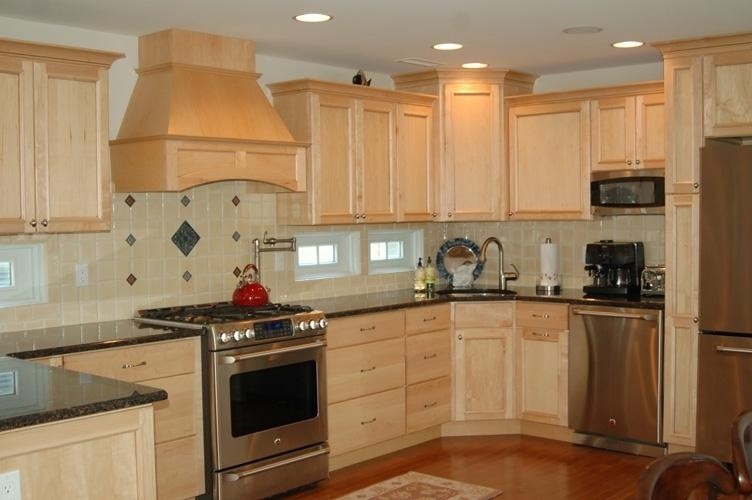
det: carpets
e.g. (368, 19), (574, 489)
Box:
(333, 469), (504, 500)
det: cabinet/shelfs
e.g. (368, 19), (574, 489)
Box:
(592, 85), (665, 173)
(266, 78), (395, 223)
(395, 90), (437, 223)
(57, 337), (205, 500)
(406, 303), (450, 449)
(442, 68), (539, 223)
(0, 39), (127, 235)
(514, 302), (569, 442)
(663, 50), (701, 447)
(507, 87), (613, 221)
(324, 301), (405, 464)
(451, 300), (513, 439)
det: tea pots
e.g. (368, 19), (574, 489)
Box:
(231, 265), (268, 307)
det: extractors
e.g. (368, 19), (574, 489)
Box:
(111, 31), (310, 196)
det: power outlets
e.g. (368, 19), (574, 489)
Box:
(1, 468), (24, 500)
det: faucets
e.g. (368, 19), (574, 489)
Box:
(480, 237), (520, 290)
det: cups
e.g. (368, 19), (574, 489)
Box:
(639, 264), (664, 297)
(424, 256), (436, 286)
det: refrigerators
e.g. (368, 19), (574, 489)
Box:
(695, 142), (752, 468)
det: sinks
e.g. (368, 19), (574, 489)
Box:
(437, 287), (516, 299)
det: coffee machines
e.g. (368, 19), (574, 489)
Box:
(582, 238), (643, 302)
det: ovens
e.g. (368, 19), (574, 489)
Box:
(195, 334), (330, 500)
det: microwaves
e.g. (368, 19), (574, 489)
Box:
(590, 170), (664, 215)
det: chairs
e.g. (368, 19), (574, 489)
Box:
(639, 451), (742, 499)
(732, 408), (752, 500)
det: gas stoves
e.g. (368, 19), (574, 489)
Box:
(132, 293), (331, 352)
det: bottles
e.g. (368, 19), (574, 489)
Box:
(412, 257), (426, 291)
(541, 237), (559, 286)
